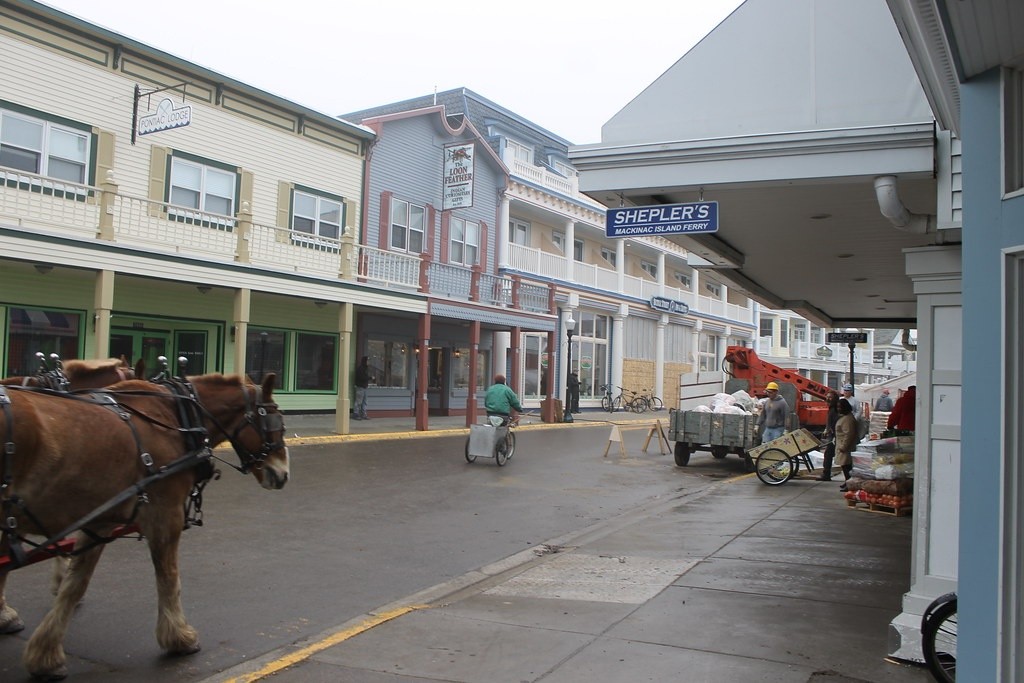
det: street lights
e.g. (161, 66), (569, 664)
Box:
(564, 314), (576, 423)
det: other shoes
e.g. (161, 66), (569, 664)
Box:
(815, 476), (831, 481)
(575, 408), (582, 413)
(840, 486), (848, 492)
(840, 483), (847, 488)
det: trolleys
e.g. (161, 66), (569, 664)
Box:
(746, 428), (833, 486)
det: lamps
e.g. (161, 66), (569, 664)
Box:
(230, 326), (235, 343)
(315, 302), (327, 309)
(35, 265), (53, 275)
(197, 287), (211, 294)
(460, 323), (471, 328)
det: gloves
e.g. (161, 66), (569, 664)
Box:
(783, 429), (789, 436)
(753, 425), (760, 435)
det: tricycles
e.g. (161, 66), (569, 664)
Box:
(465, 416), (517, 466)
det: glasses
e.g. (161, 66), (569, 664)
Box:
(766, 390), (773, 394)
(844, 389), (850, 392)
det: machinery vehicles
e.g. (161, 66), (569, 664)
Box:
(722, 345), (871, 440)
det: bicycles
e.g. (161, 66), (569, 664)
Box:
(613, 385), (663, 413)
(599, 383), (614, 414)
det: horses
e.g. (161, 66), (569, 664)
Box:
(0, 355), (291, 680)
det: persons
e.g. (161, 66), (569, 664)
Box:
(484, 375), (522, 454)
(815, 390), (844, 481)
(570, 368), (582, 414)
(835, 399), (859, 492)
(754, 382), (792, 474)
(875, 389), (893, 412)
(353, 356), (375, 420)
(841, 383), (862, 444)
(887, 385), (915, 435)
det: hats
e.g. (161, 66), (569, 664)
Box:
(573, 368), (579, 371)
(883, 389), (890, 394)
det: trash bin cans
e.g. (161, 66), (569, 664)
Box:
(540, 398), (563, 423)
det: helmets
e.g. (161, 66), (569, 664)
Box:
(843, 384), (852, 390)
(765, 382), (779, 391)
